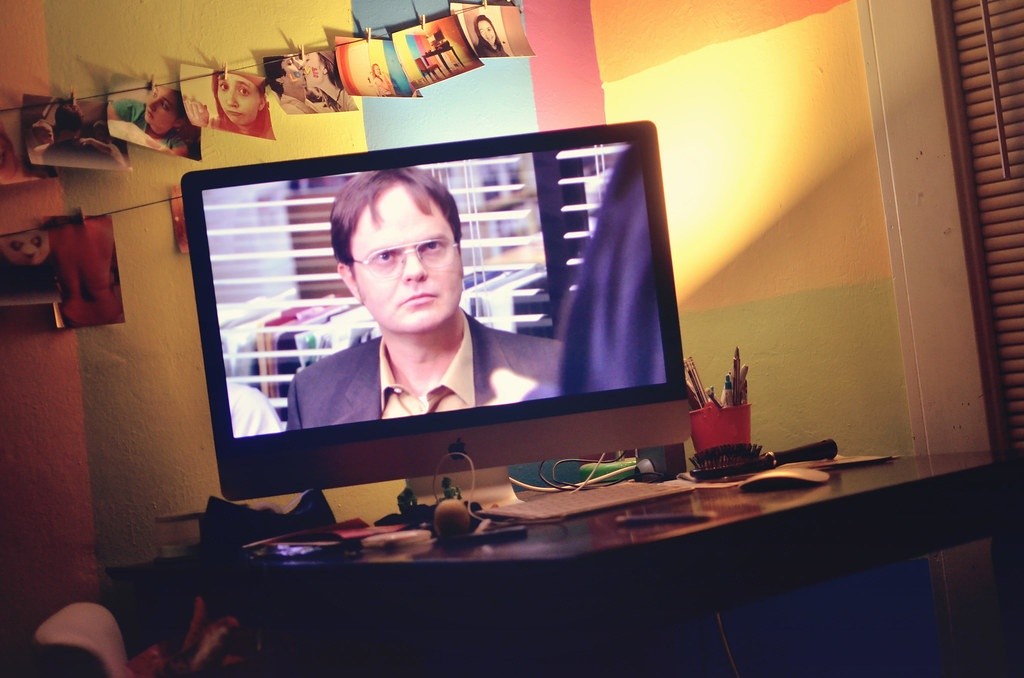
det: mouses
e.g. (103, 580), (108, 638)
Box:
(739, 467), (828, 493)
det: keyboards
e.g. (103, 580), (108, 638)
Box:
(482, 482), (697, 521)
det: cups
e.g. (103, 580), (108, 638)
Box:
(686, 404), (751, 459)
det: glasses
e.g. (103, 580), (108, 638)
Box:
(353, 239), (458, 281)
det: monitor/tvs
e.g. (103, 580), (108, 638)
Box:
(184, 127), (691, 511)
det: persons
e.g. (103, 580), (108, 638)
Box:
(264, 52), (359, 114)
(107, 85), (188, 157)
(366, 63), (395, 97)
(183, 70), (276, 140)
(474, 15), (509, 57)
(286, 167), (566, 434)
(31, 104), (129, 170)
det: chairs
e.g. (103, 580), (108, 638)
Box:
(34, 602), (128, 678)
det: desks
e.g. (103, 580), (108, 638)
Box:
(103, 452), (1024, 678)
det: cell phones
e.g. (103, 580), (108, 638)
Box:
(433, 525), (527, 550)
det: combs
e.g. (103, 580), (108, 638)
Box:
(686, 439), (838, 479)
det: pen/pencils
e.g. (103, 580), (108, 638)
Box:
(684, 345), (749, 410)
(432, 526), (529, 547)
(615, 513), (712, 526)
